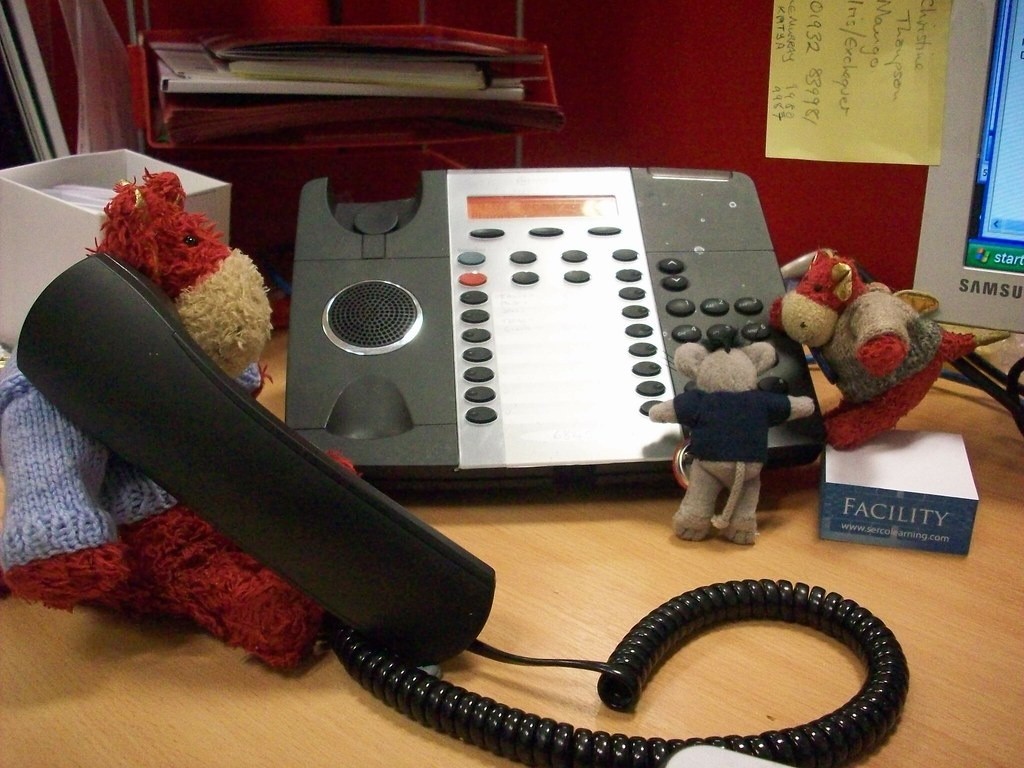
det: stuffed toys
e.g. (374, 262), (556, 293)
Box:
(0, 168), (359, 671)
(649, 342), (815, 544)
(769, 250), (1008, 450)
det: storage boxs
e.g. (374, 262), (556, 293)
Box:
(0, 148), (234, 350)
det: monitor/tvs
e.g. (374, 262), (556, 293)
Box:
(912, 1), (1024, 331)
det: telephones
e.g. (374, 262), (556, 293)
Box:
(14, 251), (496, 664)
